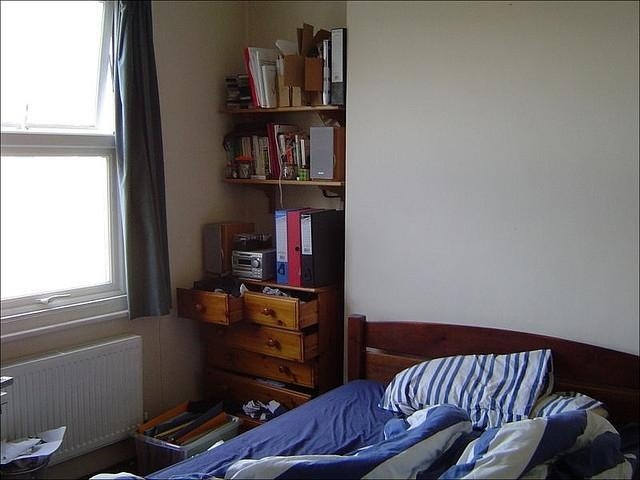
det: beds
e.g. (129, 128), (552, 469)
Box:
(90, 314), (640, 480)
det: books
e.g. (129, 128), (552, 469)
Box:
(221, 44), (310, 180)
(139, 399), (234, 447)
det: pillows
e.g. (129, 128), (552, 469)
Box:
(531, 391), (608, 425)
(378, 349), (554, 431)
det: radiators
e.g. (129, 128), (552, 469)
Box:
(0, 333), (143, 466)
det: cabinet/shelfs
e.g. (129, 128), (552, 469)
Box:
(178, 279), (318, 435)
(216, 104), (344, 187)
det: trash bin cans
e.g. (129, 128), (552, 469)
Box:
(0, 437), (51, 480)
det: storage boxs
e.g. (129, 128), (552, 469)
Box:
(131, 414), (243, 473)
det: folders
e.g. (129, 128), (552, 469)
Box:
(275, 209), (345, 288)
